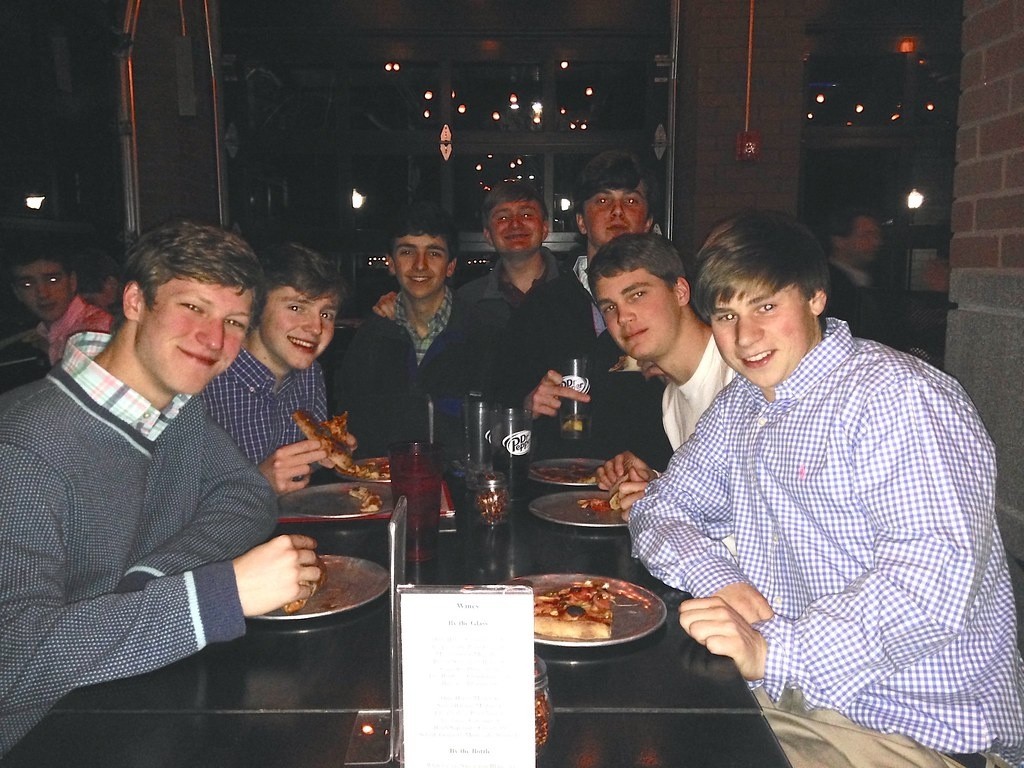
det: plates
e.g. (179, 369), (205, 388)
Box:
(282, 482), (395, 519)
(500, 572), (667, 648)
(248, 555), (389, 621)
(527, 458), (607, 486)
(335, 455), (391, 482)
(528, 490), (628, 528)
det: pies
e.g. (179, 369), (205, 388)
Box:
(608, 355), (640, 371)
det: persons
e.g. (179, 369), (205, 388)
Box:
(77, 244), (120, 305)
(210, 237), (348, 498)
(826, 211), (894, 344)
(590, 233), (734, 521)
(629, 212), (1024, 767)
(371, 150), (674, 472)
(333, 204), (494, 456)
(0, 225), (321, 754)
(3, 234), (117, 372)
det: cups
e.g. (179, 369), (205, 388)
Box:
(462, 400), (502, 476)
(557, 355), (594, 439)
(386, 441), (444, 560)
(489, 408), (536, 493)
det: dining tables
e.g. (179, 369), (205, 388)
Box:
(0, 457), (792, 768)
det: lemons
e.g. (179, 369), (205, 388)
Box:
(562, 421), (582, 431)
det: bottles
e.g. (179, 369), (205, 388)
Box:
(474, 475), (514, 526)
(534, 654), (552, 756)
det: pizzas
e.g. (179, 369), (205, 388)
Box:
(528, 465), (598, 482)
(534, 586), (613, 640)
(348, 485), (382, 513)
(284, 556), (328, 613)
(290, 410), (355, 473)
(337, 456), (390, 480)
